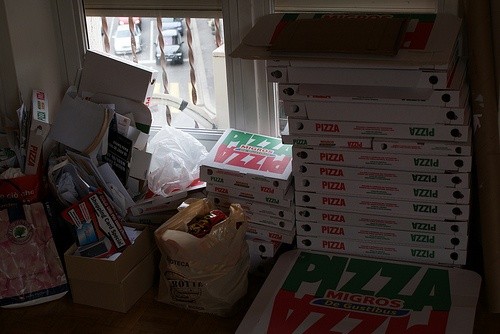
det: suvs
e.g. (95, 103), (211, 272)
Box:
(152, 30), (185, 65)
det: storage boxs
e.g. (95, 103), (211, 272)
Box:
(0, 12), (478, 334)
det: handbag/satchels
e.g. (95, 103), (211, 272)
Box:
(0, 180), (69, 309)
(154, 197), (249, 315)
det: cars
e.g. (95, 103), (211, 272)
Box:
(118, 17), (142, 26)
(111, 23), (142, 54)
(161, 18), (184, 36)
